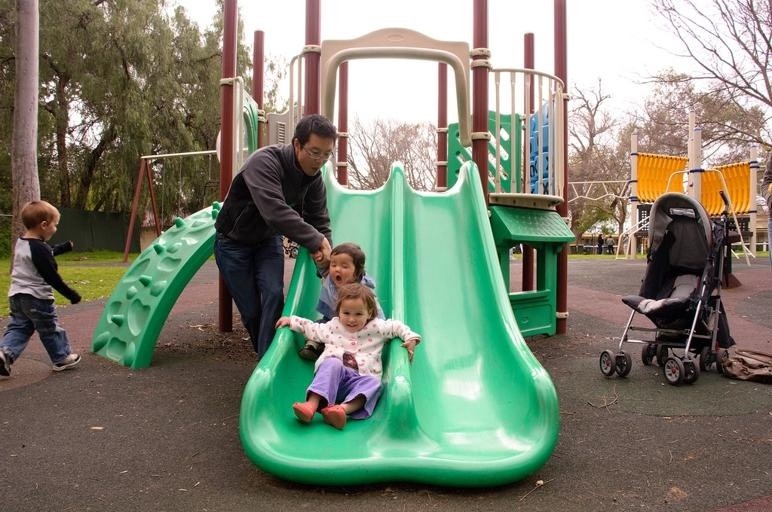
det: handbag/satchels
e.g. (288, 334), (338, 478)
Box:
(722, 347), (772, 381)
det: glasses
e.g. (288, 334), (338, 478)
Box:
(301, 144), (335, 160)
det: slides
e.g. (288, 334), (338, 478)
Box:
(239, 159), (560, 490)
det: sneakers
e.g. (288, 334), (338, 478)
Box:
(298, 343), (325, 360)
(0, 348), (12, 376)
(292, 401), (347, 429)
(52, 354), (81, 371)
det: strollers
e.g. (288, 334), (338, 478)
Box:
(601, 191), (734, 387)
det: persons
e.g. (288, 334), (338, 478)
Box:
(214, 113), (340, 363)
(607, 235), (615, 255)
(597, 234), (604, 254)
(297, 242), (387, 362)
(760, 150), (771, 272)
(0, 200), (84, 378)
(274, 282), (422, 431)
(623, 233), (628, 256)
